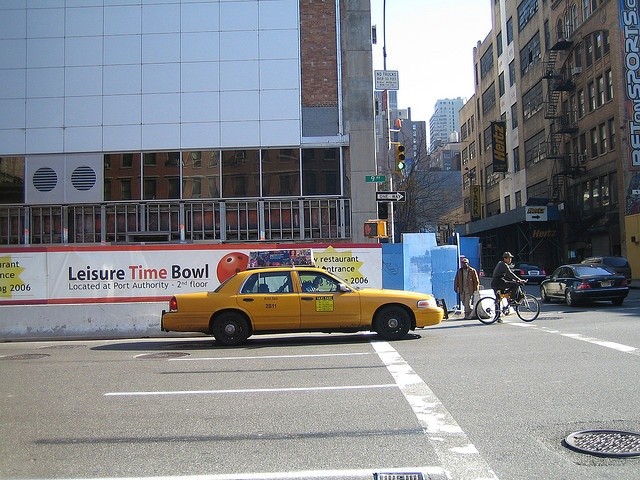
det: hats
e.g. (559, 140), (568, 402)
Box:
(502, 251), (514, 258)
(461, 258), (469, 264)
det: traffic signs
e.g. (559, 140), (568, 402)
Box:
(375, 191), (406, 203)
(364, 175), (386, 182)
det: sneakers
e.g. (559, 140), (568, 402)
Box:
(510, 299), (520, 306)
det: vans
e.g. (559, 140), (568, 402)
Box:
(580, 256), (632, 285)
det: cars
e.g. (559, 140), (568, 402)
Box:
(161, 267), (444, 346)
(512, 262), (546, 285)
(539, 263), (629, 307)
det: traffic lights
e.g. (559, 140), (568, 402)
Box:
(395, 145), (406, 173)
(363, 219), (387, 239)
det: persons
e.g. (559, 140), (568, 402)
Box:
(302, 276), (323, 292)
(453, 258), (480, 320)
(258, 282), (289, 293)
(491, 252), (526, 323)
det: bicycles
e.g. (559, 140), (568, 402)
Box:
(475, 279), (540, 325)
